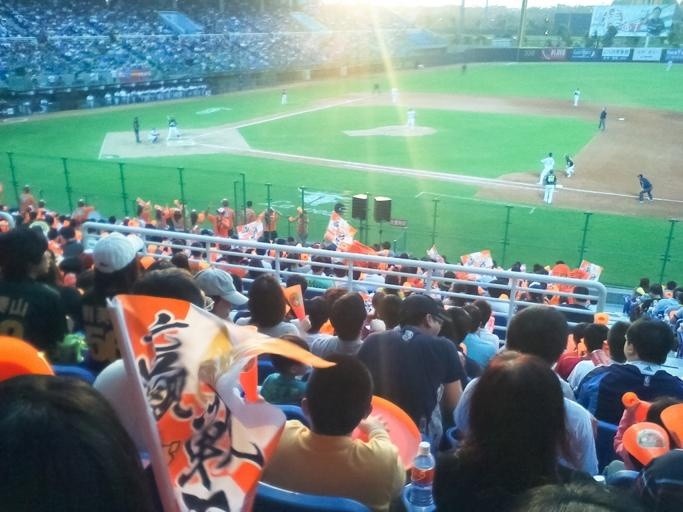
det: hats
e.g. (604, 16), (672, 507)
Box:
(193, 266), (249, 306)
(93, 231), (143, 273)
(398, 294), (453, 325)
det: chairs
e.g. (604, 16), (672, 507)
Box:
(45, 357), (646, 512)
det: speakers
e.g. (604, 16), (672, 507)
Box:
(374, 196), (391, 222)
(352, 194), (368, 221)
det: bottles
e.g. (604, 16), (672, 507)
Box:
(408, 441), (436, 506)
(484, 311), (495, 333)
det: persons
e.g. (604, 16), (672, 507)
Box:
(1, 186), (683, 512)
(597, 108), (607, 130)
(573, 88), (581, 106)
(565, 155), (575, 177)
(638, 175), (653, 204)
(148, 128), (160, 144)
(538, 153), (554, 185)
(406, 109), (415, 129)
(166, 116), (180, 141)
(642, 6), (666, 38)
(1, 0), (466, 117)
(667, 57), (673, 71)
(544, 170), (556, 203)
(133, 117), (141, 143)
